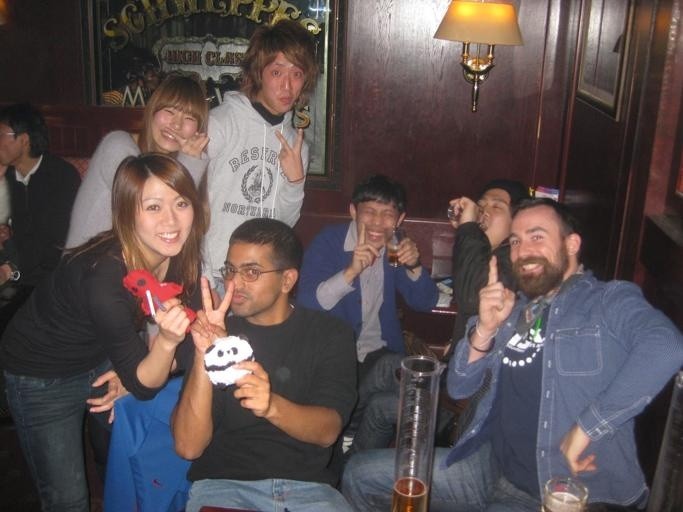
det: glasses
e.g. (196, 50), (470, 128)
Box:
(219, 266), (287, 282)
(1, 132), (17, 139)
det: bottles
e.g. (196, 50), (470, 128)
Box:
(390, 355), (442, 512)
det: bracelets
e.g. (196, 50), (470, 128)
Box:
(288, 174), (304, 185)
(6, 260), (21, 283)
(467, 326), (497, 356)
(7, 224), (14, 242)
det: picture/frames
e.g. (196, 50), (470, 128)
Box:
(575, 1), (636, 123)
(80, 1), (349, 192)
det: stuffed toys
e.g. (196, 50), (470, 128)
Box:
(119, 268), (198, 335)
(202, 333), (257, 394)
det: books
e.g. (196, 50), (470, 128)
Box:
(535, 185), (560, 203)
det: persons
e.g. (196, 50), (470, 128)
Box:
(339, 177), (530, 472)
(338, 197), (683, 510)
(0, 162), (13, 244)
(168, 217), (360, 512)
(293, 175), (441, 389)
(0, 103), (82, 328)
(195, 18), (324, 316)
(59, 70), (212, 265)
(0, 152), (208, 512)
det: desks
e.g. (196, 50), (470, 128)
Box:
(398, 277), (459, 351)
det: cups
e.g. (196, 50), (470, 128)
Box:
(447, 205), (489, 232)
(385, 227), (405, 267)
(543, 477), (589, 512)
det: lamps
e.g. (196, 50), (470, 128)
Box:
(433, 0), (525, 112)
